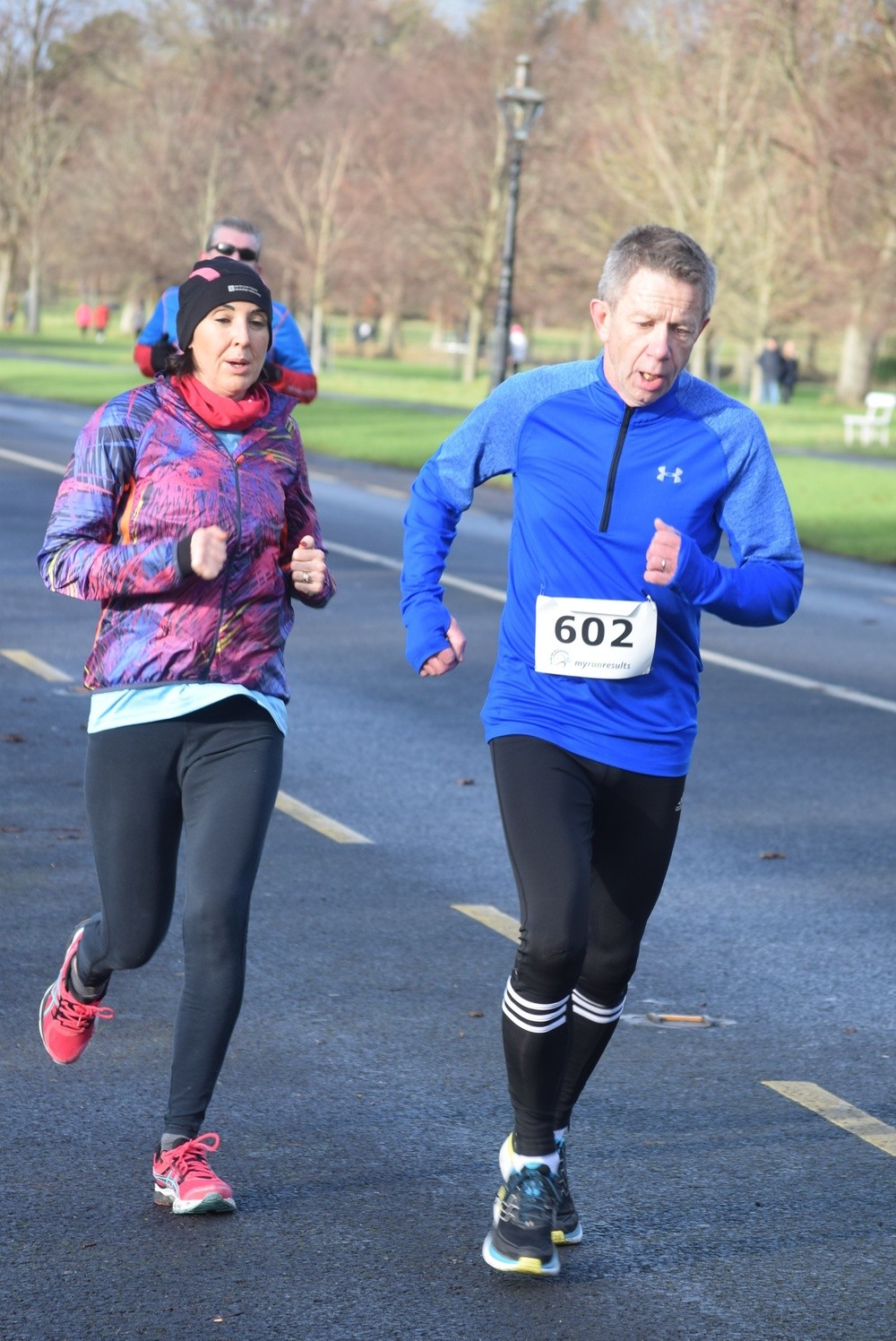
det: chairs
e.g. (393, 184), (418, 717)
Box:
(841, 392), (895, 448)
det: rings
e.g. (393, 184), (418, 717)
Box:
(302, 571), (309, 583)
(661, 559), (666, 572)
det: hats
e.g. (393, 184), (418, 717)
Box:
(177, 256), (272, 355)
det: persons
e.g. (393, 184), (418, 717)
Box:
(398, 221), (805, 1279)
(753, 330), (799, 404)
(506, 316), (529, 377)
(134, 217), (318, 424)
(76, 298), (109, 343)
(35, 257), (340, 1217)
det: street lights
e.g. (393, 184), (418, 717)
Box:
(489, 51), (544, 391)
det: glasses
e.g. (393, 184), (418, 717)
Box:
(208, 242), (257, 261)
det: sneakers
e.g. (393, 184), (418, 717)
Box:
(499, 1131), (582, 1245)
(153, 1133), (236, 1214)
(483, 1162), (560, 1274)
(39, 918), (114, 1065)
